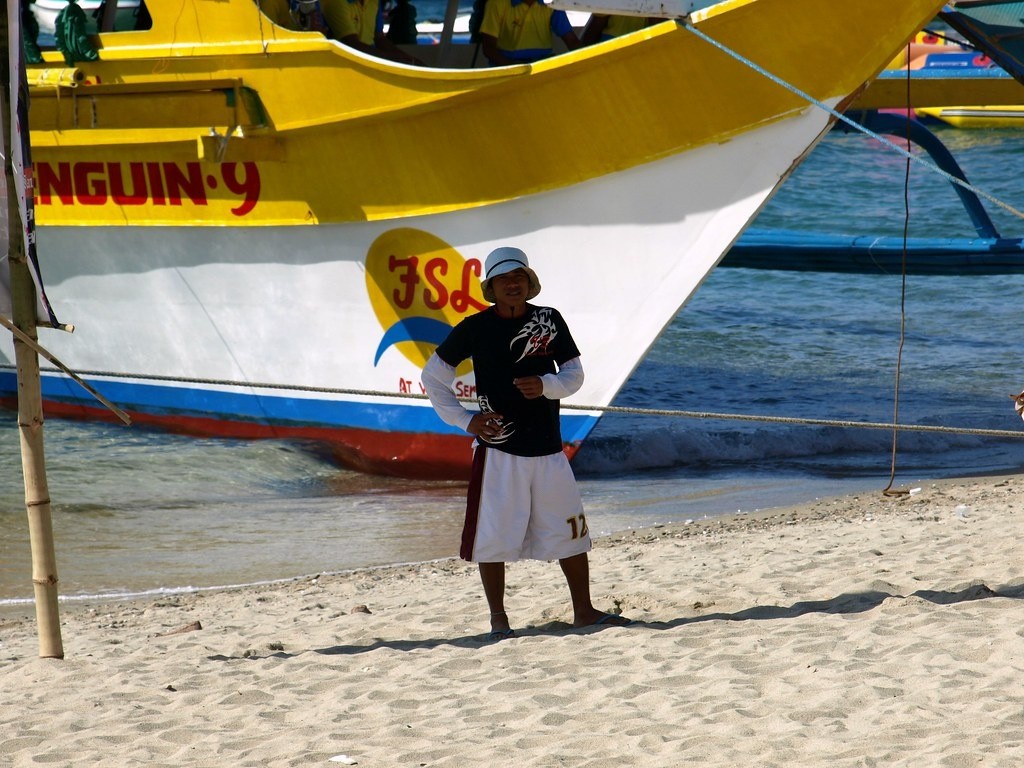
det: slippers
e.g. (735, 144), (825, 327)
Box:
(487, 629), (514, 643)
(596, 613), (635, 626)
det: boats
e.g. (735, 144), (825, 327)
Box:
(1, 1), (957, 482)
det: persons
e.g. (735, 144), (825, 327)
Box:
(470, 0), (488, 43)
(479, 0), (582, 65)
(21, 0), (39, 40)
(257, 0), (398, 58)
(422, 247), (632, 641)
(388, 0), (417, 44)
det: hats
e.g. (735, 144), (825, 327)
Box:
(480, 247), (541, 303)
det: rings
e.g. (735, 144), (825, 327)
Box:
(486, 421), (489, 425)
